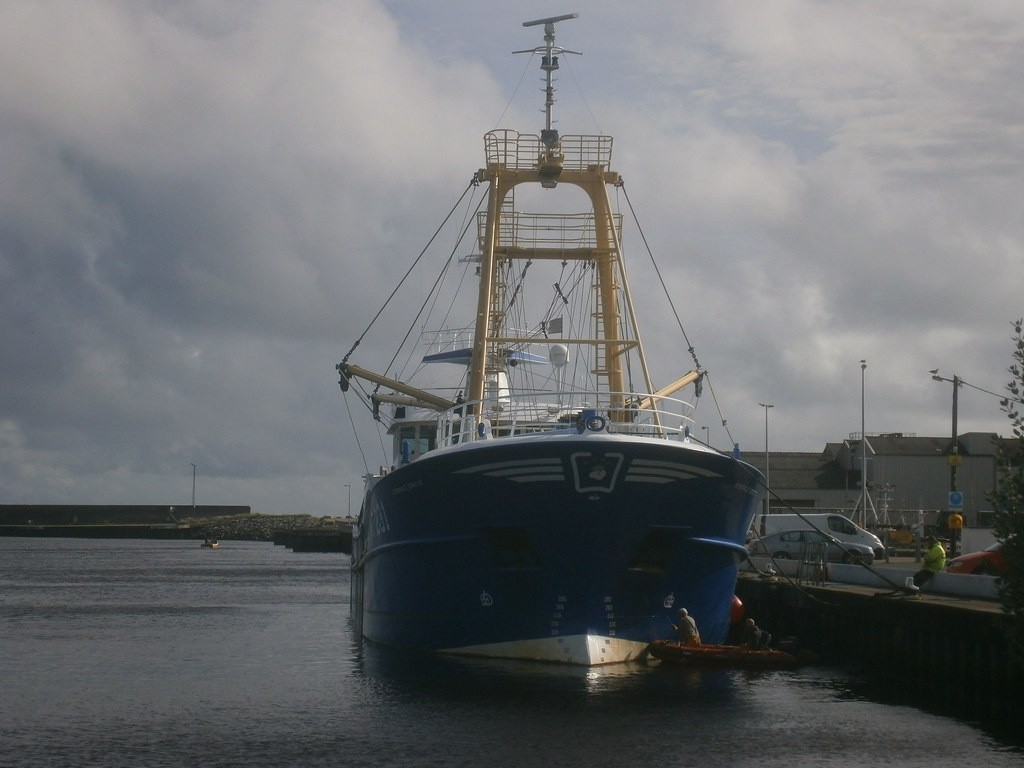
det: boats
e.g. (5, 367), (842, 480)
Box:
(646, 639), (797, 669)
(201, 539), (219, 548)
(333, 13), (768, 668)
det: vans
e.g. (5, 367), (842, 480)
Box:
(755, 512), (885, 559)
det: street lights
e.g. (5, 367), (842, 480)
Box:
(702, 426), (710, 449)
(192, 463), (196, 507)
(759, 403), (774, 513)
(861, 364), (867, 528)
(930, 374), (959, 491)
(345, 484), (350, 526)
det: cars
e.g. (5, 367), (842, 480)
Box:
(945, 533), (1019, 575)
(748, 529), (875, 565)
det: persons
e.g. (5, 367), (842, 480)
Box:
(670, 608), (765, 651)
(911, 535), (946, 587)
